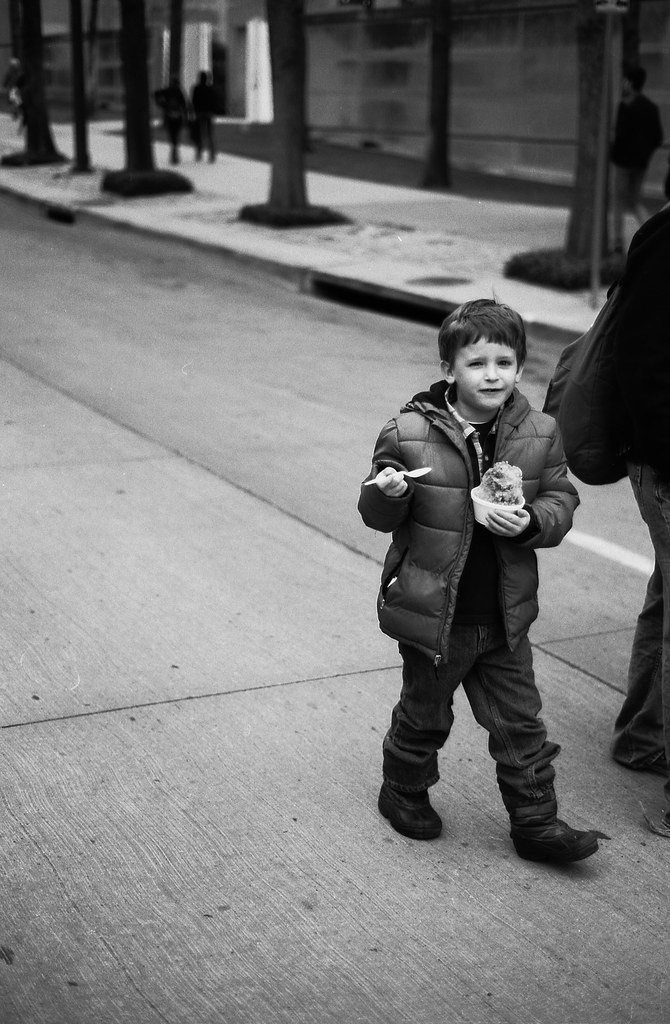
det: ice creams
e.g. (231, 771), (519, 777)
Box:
(471, 460), (525, 525)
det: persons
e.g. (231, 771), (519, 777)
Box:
(357, 300), (598, 863)
(610, 172), (670, 824)
(190, 71), (216, 162)
(615, 64), (663, 254)
(154, 82), (186, 163)
(4, 58), (27, 134)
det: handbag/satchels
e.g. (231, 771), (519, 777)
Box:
(542, 280), (630, 485)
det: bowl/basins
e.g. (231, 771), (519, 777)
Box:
(470, 486), (526, 526)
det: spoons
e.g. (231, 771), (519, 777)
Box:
(364, 466), (432, 487)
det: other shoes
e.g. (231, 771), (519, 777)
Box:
(661, 812), (670, 830)
(647, 754), (670, 777)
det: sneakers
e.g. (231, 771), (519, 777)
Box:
(379, 781), (442, 840)
(513, 825), (599, 864)
